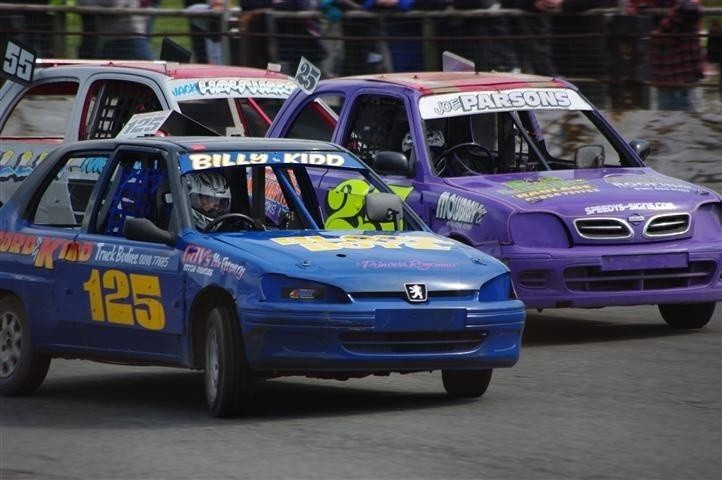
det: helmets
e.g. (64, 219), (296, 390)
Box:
(402, 128), (445, 152)
(183, 173), (233, 231)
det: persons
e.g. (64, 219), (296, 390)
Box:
(0, 1), (162, 58)
(183, 0), (721, 110)
(168, 171), (267, 233)
(401, 126), (456, 177)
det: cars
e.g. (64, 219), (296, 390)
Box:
(1, 58), (377, 210)
(245, 72), (722, 330)
(0, 135), (525, 421)
